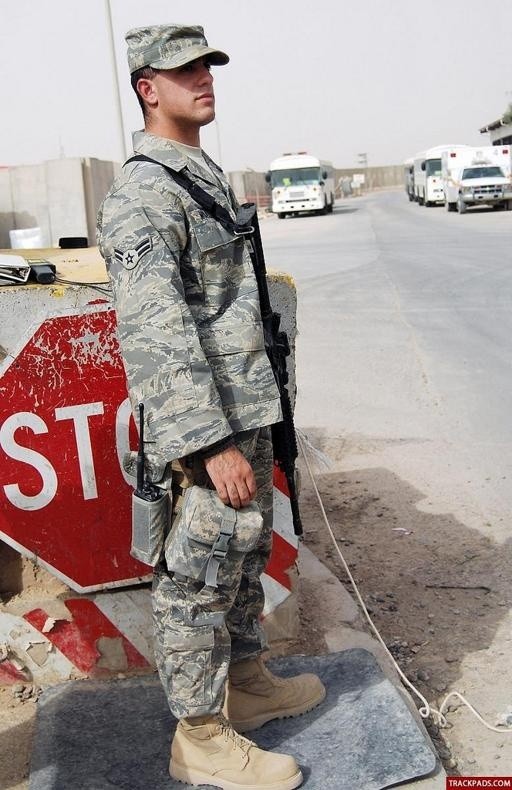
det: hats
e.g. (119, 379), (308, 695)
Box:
(124, 25), (230, 76)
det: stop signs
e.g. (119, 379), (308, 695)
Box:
(0, 307), (152, 600)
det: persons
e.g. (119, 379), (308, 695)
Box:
(96, 25), (328, 790)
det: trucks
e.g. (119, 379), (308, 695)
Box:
(402, 142), (512, 217)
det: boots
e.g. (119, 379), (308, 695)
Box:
(168, 711), (303, 790)
(223, 654), (327, 733)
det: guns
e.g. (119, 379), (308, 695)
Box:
(236, 203), (304, 535)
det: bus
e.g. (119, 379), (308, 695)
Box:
(264, 151), (340, 219)
